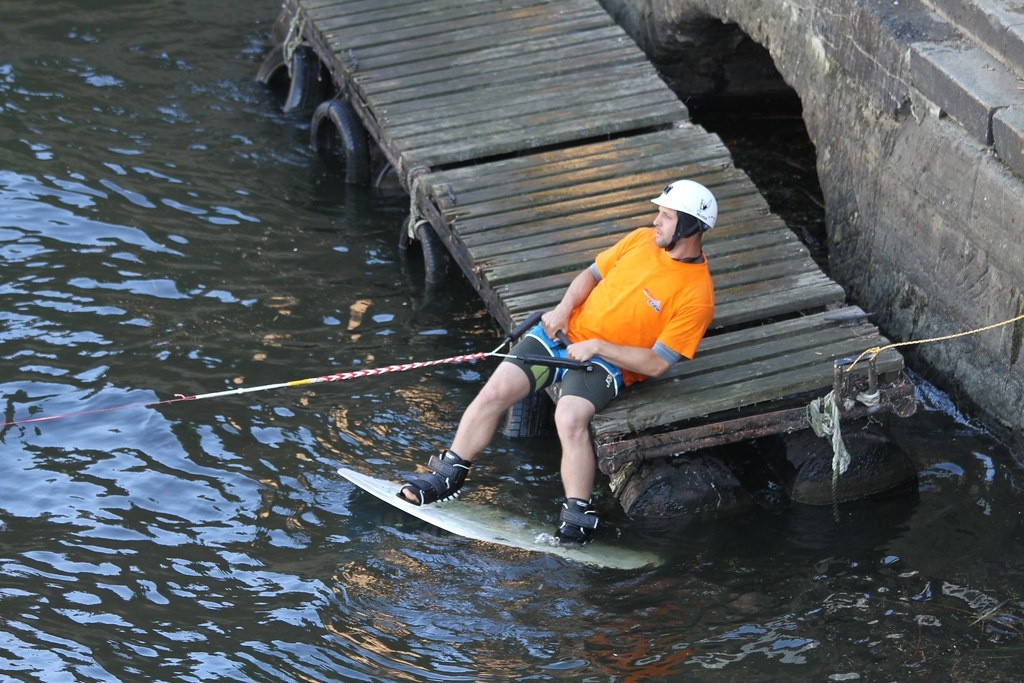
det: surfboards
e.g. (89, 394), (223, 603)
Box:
(335, 464), (665, 572)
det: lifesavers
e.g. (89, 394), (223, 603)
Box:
(253, 39), (320, 117)
(399, 214), (451, 287)
(306, 95), (372, 189)
(503, 385), (541, 441)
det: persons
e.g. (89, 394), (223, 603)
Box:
(399, 180), (719, 544)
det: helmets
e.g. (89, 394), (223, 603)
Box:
(651, 179), (718, 228)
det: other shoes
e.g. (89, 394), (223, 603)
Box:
(552, 497), (600, 548)
(396, 449), (472, 509)
